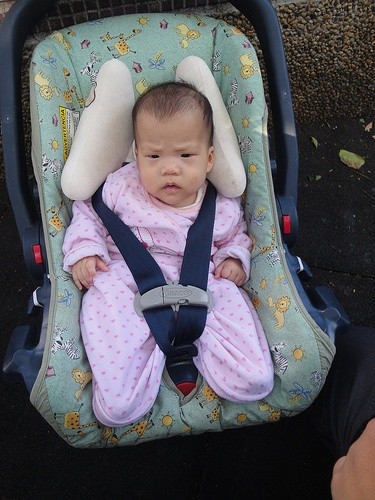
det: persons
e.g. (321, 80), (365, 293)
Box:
(61, 80), (276, 426)
(328, 419), (375, 499)
